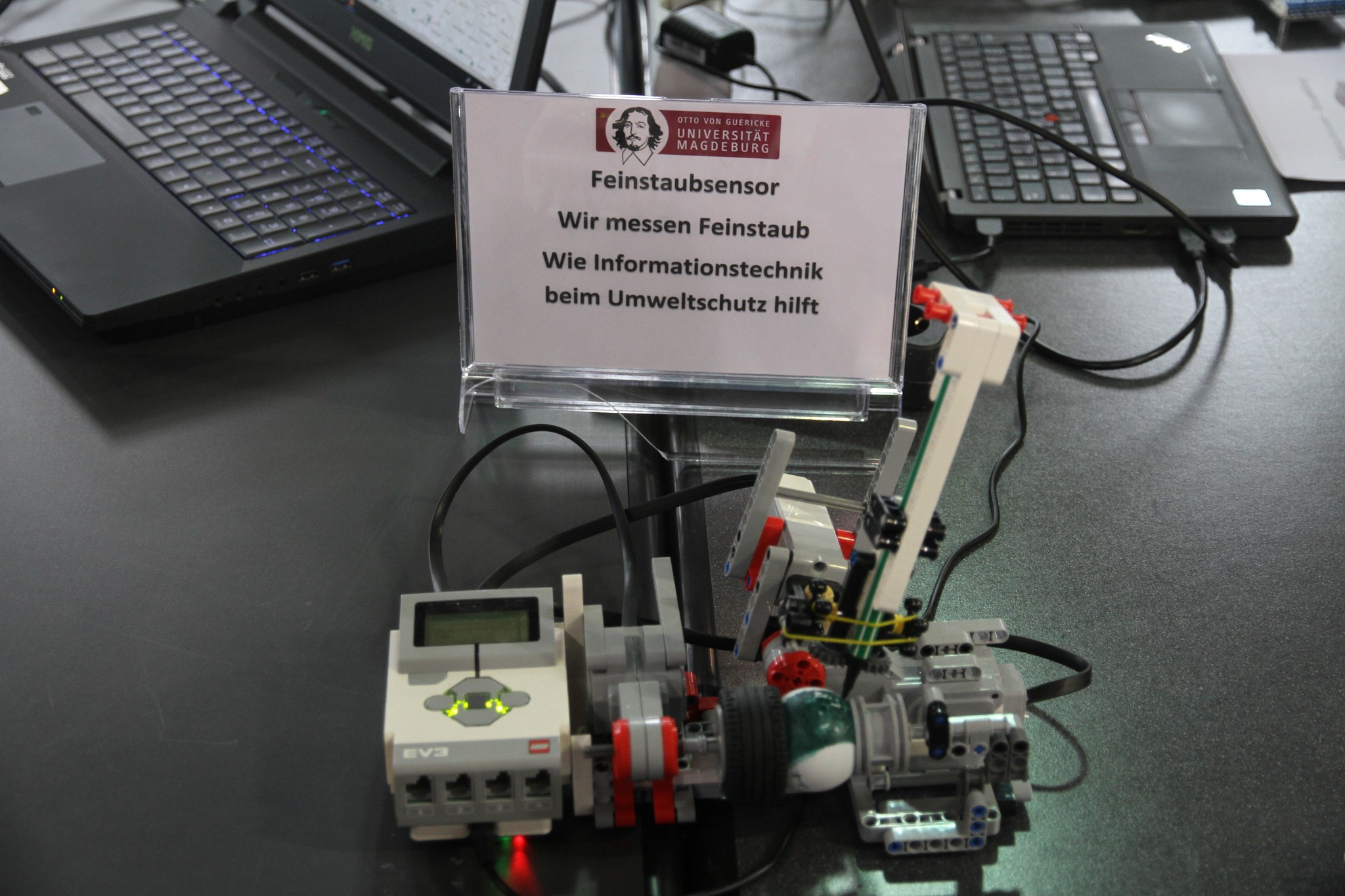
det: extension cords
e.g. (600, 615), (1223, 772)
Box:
(646, 1), (731, 102)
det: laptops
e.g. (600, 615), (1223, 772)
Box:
(0, 0), (558, 334)
(849, 0), (1302, 240)
